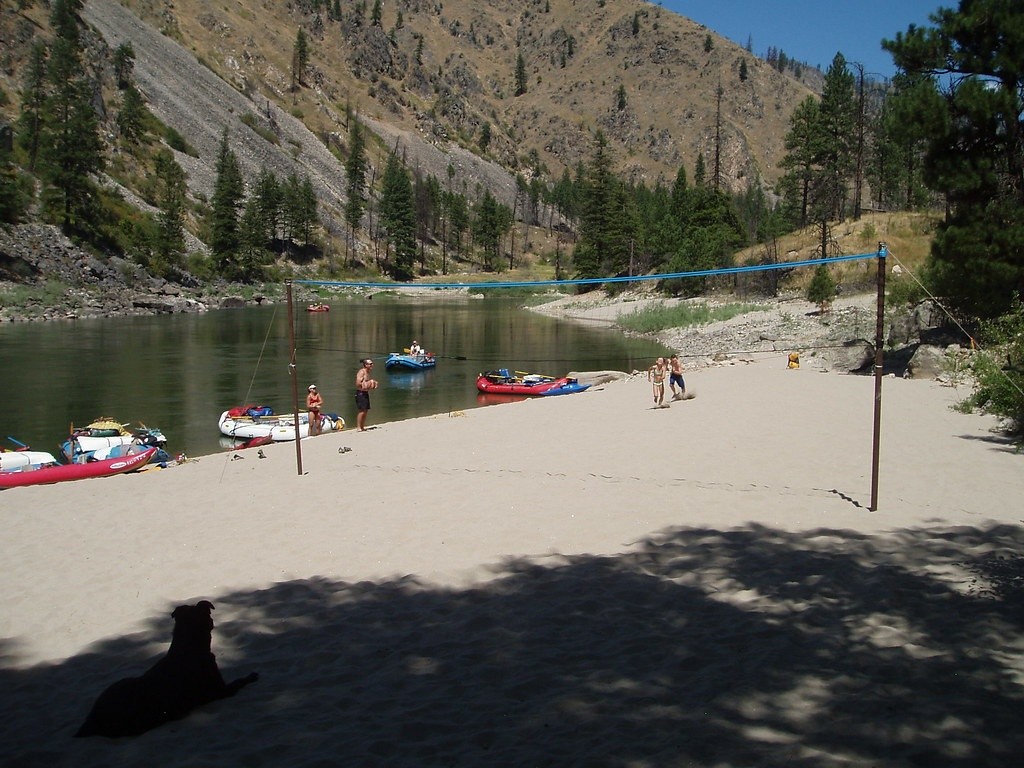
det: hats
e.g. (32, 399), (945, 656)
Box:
(308, 384), (316, 391)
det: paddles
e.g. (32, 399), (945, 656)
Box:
(413, 355), (466, 359)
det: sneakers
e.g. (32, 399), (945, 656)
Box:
(338, 446), (352, 454)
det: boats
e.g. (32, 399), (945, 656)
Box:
(0, 446), (159, 490)
(385, 349), (436, 369)
(476, 367), (593, 395)
(60, 420), (168, 467)
(0, 449), (57, 473)
(305, 302), (329, 311)
(218, 410), (346, 440)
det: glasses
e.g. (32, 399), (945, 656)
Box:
(310, 387), (316, 390)
(367, 362), (373, 365)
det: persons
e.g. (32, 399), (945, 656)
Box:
(410, 340), (420, 359)
(647, 357), (666, 406)
(306, 384), (324, 436)
(354, 357), (378, 432)
(666, 354), (686, 399)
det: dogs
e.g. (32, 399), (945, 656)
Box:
(72, 600), (259, 740)
